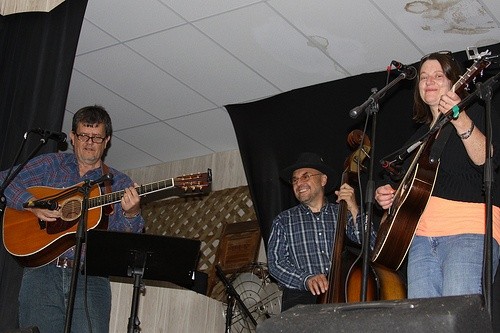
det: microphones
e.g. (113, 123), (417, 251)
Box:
(392, 60), (417, 80)
(23, 201), (58, 210)
(379, 159), (405, 180)
(31, 127), (67, 144)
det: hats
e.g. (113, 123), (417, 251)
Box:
(290, 152), (329, 176)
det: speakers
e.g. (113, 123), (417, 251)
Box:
(254, 294), (493, 333)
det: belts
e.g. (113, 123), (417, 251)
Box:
(50, 257), (84, 269)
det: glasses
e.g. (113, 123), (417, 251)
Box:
(421, 50), (455, 63)
(288, 173), (323, 185)
(74, 130), (106, 143)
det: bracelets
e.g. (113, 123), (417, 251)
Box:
(458, 121), (475, 139)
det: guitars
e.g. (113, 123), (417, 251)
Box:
(2, 172), (210, 269)
(370, 56), (493, 271)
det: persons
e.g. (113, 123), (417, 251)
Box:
(0, 104), (145, 333)
(267, 152), (377, 313)
(374, 50), (500, 299)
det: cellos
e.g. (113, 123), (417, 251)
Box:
(316, 130), (409, 303)
